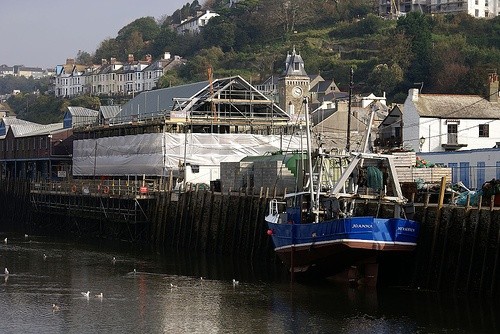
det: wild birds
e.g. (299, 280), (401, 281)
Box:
(111, 256), (116, 264)
(127, 269), (137, 275)
(232, 279), (240, 284)
(4, 238), (8, 245)
(80, 290), (91, 298)
(52, 303), (60, 310)
(25, 234), (28, 237)
(94, 292), (103, 298)
(4, 268), (10, 274)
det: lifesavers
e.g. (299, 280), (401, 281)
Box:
(104, 186), (109, 194)
(71, 185), (76, 192)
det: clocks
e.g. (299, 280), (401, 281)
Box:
(291, 85), (303, 99)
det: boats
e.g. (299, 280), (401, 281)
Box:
(264, 97), (420, 292)
